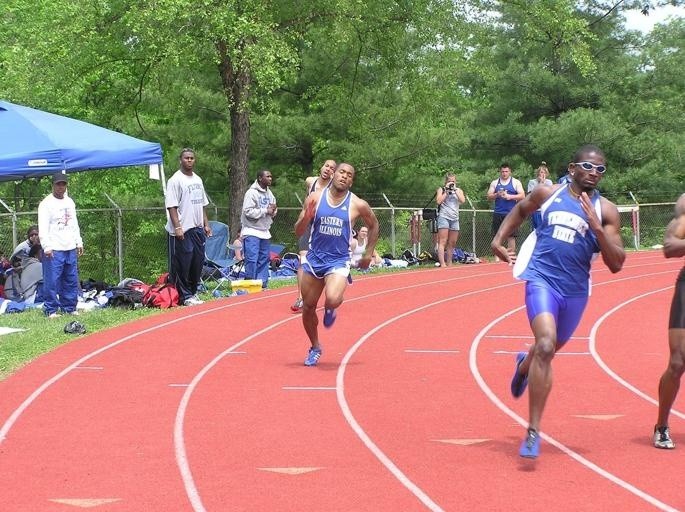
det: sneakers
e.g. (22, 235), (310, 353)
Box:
(347, 273), (353, 285)
(184, 293), (206, 306)
(510, 351), (528, 398)
(519, 427), (542, 458)
(289, 296), (303, 312)
(652, 424), (675, 449)
(303, 345), (322, 367)
(46, 310), (80, 319)
(322, 304), (337, 327)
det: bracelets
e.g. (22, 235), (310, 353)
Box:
(176, 226), (181, 229)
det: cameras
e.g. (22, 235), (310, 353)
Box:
(449, 183), (455, 190)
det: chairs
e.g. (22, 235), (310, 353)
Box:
(200, 221), (241, 301)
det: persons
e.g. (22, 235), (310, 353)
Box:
(527, 165), (553, 230)
(20, 244), (43, 303)
(37, 173), (84, 318)
(164, 149), (212, 306)
(490, 144), (625, 458)
(652, 192), (685, 449)
(558, 161), (574, 184)
(291, 159), (337, 311)
(351, 225), (378, 268)
(294, 162), (379, 366)
(487, 164), (525, 266)
(230, 226), (245, 276)
(436, 174), (465, 268)
(9, 225), (38, 260)
(241, 168), (277, 289)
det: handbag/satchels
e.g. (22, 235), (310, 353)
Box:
(77, 271), (180, 311)
(375, 245), (481, 270)
(422, 187), (445, 220)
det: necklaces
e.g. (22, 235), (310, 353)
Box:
(568, 183), (596, 199)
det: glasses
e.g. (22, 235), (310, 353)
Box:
(573, 161), (608, 175)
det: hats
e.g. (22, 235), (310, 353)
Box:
(52, 173), (68, 185)
